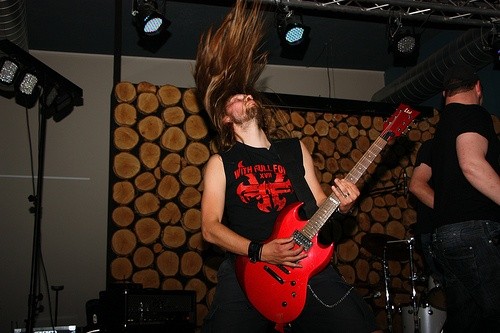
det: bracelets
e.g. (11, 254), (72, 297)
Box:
(248, 240), (264, 263)
(337, 207), (354, 215)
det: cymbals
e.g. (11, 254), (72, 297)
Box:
(361, 233), (410, 261)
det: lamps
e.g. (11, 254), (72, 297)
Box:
(131, 0), (172, 38)
(387, 11), (419, 54)
(0, 38), (84, 333)
(274, 0), (310, 45)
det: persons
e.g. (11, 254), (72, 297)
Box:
(192, 0), (374, 333)
(409, 63), (500, 333)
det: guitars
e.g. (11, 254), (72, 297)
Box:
(233, 102), (422, 333)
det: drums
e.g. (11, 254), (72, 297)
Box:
(428, 274), (447, 312)
(401, 301), (448, 333)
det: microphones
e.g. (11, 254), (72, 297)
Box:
(403, 169), (408, 200)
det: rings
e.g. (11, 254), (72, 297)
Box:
(345, 193), (350, 197)
